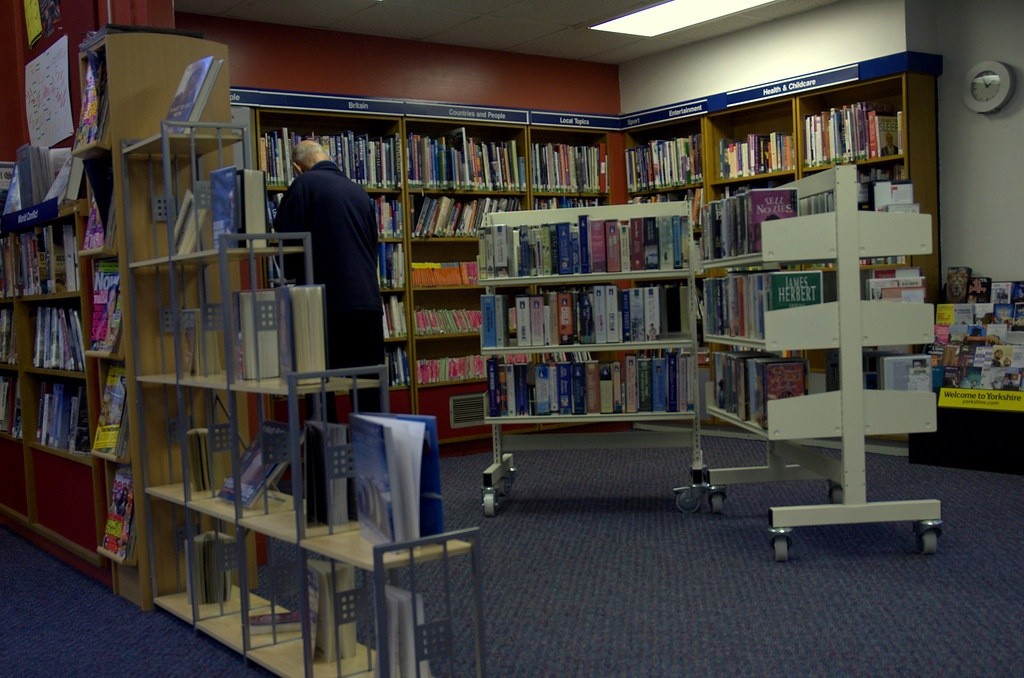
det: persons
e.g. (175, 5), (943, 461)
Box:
(263, 140), (387, 412)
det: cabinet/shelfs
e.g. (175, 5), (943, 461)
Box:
(2, 0), (1024, 678)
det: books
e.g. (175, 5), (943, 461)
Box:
(208, 166), (449, 678)
(1, 42), (223, 570)
(402, 99), (1024, 433)
(258, 126), (412, 388)
